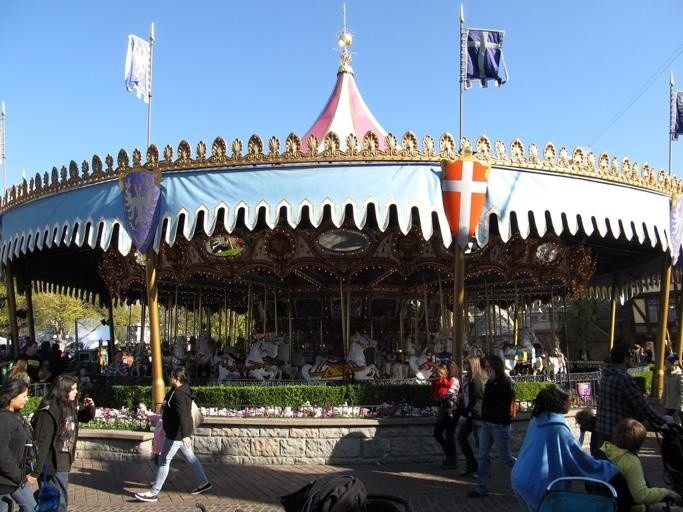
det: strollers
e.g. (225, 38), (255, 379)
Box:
(648, 420), (680, 492)
(531, 475), (617, 512)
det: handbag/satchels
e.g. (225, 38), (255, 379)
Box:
(190, 400), (204, 430)
(34, 461), (60, 512)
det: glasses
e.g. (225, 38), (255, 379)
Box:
(462, 361), (468, 365)
(168, 374), (174, 378)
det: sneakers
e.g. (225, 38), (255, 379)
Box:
(439, 464), (490, 478)
(467, 490), (488, 497)
(134, 492), (158, 502)
(190, 482), (212, 495)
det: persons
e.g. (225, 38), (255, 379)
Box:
(505, 381), (621, 511)
(431, 358), (462, 470)
(464, 354), (517, 498)
(453, 354), (483, 477)
(132, 366), (212, 502)
(585, 416), (682, 511)
(0, 378), (41, 512)
(630, 338), (678, 365)
(588, 341), (677, 460)
(659, 352), (683, 426)
(30, 374), (97, 512)
(0, 330), (132, 387)
(429, 365), (458, 419)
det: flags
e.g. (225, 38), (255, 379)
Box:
(670, 90), (683, 138)
(122, 33), (154, 106)
(458, 23), (509, 92)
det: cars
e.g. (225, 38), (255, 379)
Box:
(64, 348), (96, 364)
